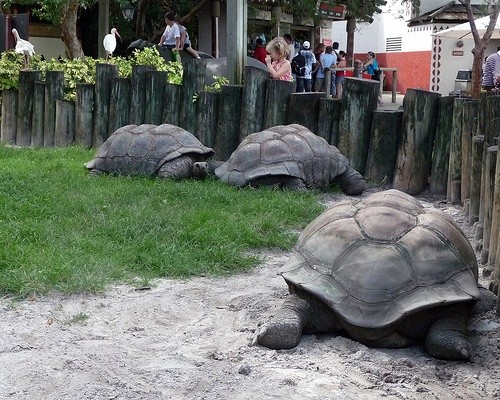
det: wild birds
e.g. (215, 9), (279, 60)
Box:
(12, 28), (35, 72)
(103, 28), (121, 64)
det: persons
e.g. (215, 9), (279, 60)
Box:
(310, 52), (320, 94)
(293, 40), (316, 92)
(482, 49), (500, 92)
(264, 36), (292, 82)
(173, 14), (201, 60)
(253, 38), (268, 66)
(313, 42), (326, 63)
(283, 33), (295, 71)
(313, 46), (333, 92)
(157, 11), (180, 65)
(335, 50), (347, 98)
(364, 50), (384, 106)
(481, 57), (488, 73)
(331, 41), (340, 98)
